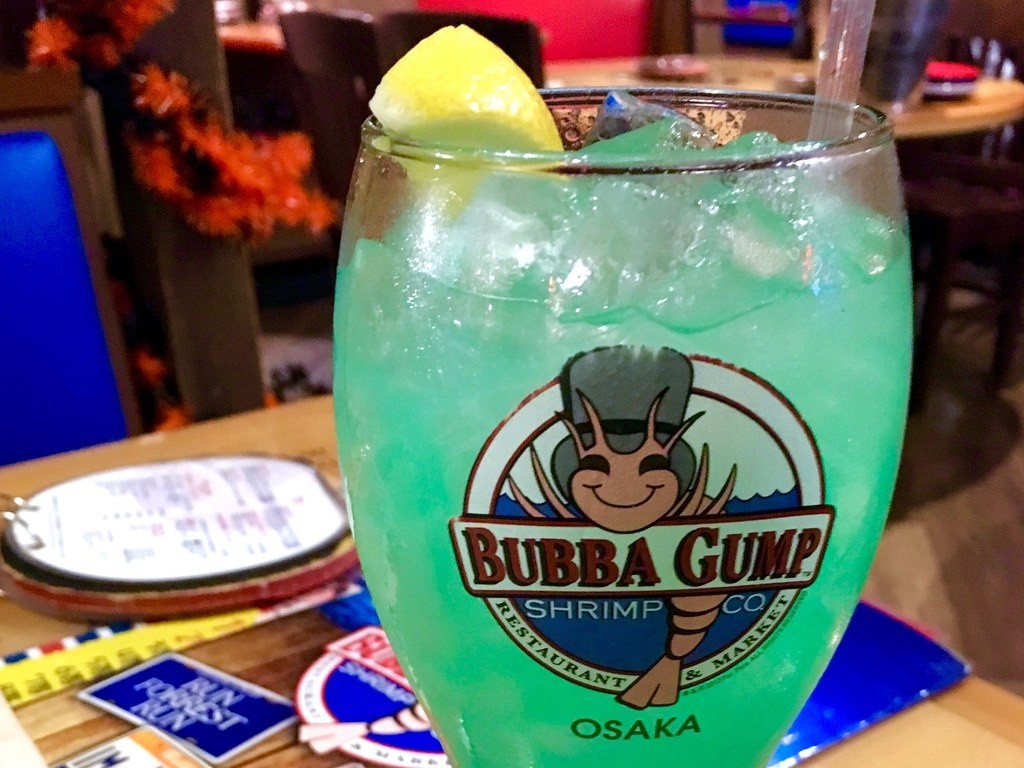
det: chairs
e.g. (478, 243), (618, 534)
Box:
(285, 5), (542, 244)
(903, 38), (1024, 422)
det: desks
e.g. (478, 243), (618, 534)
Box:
(547, 52), (1024, 371)
(0, 391), (1024, 768)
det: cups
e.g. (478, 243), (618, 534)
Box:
(333, 84), (913, 768)
(852, 0), (948, 117)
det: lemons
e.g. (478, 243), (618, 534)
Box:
(367, 22), (567, 199)
